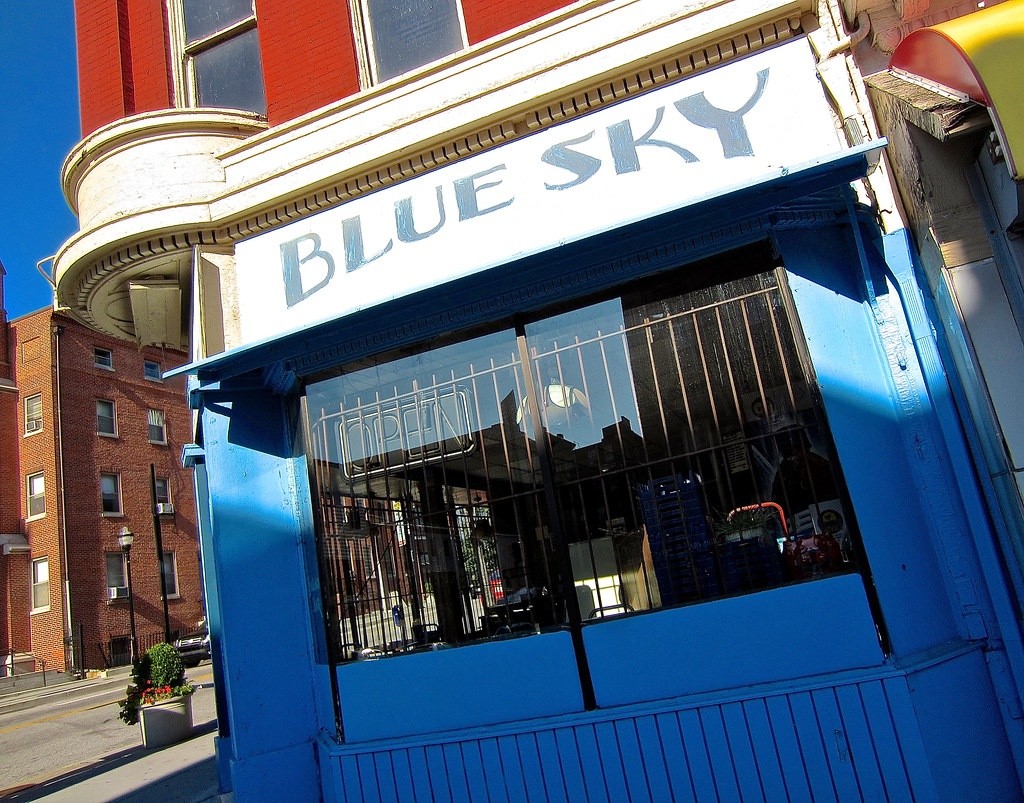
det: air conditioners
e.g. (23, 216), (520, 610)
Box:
(108, 586), (129, 599)
(157, 503), (175, 514)
(26, 420), (40, 430)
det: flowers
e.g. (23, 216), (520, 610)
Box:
(118, 643), (198, 722)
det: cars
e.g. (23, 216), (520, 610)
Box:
(488, 569), (504, 605)
(469, 577), (483, 599)
(170, 619), (212, 670)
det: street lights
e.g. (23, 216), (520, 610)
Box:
(116, 525), (139, 665)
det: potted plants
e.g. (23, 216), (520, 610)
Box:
(711, 496), (782, 546)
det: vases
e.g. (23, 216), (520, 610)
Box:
(139, 694), (195, 747)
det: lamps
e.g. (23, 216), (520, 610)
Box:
(340, 496), (381, 540)
(472, 496), (496, 541)
(516, 366), (590, 426)
